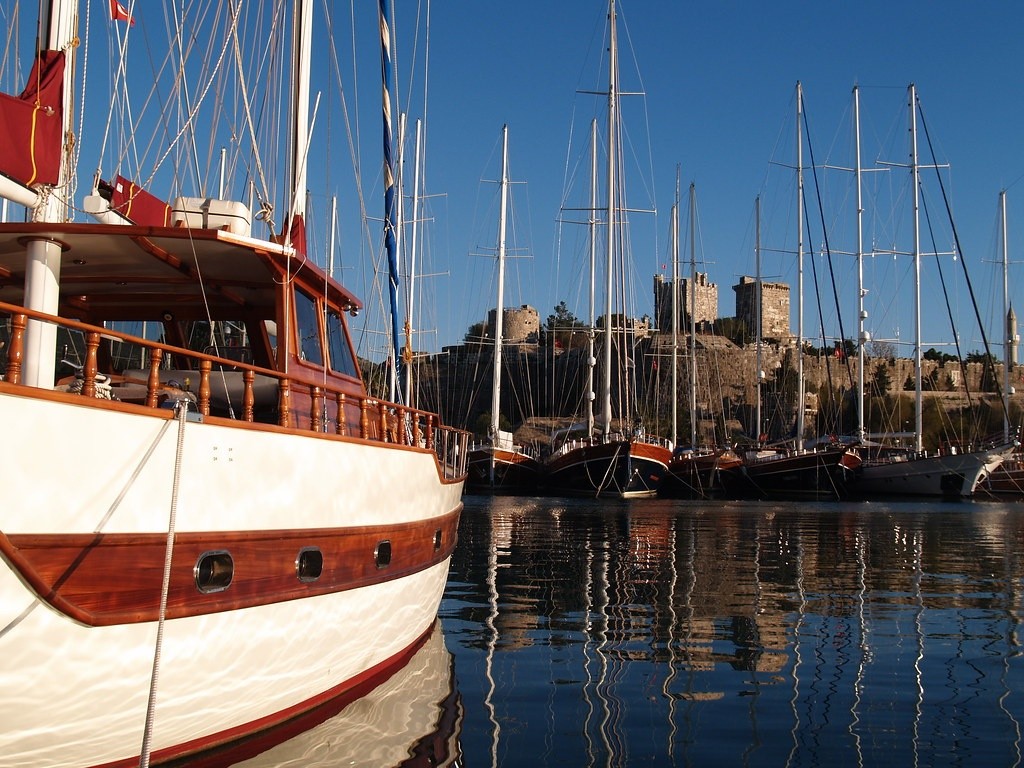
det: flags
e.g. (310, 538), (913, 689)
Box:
(661, 263), (667, 270)
(110, 0), (134, 25)
(834, 347), (844, 357)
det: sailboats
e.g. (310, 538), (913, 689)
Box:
(2, 2), (475, 768)
(454, 4), (1024, 503)
